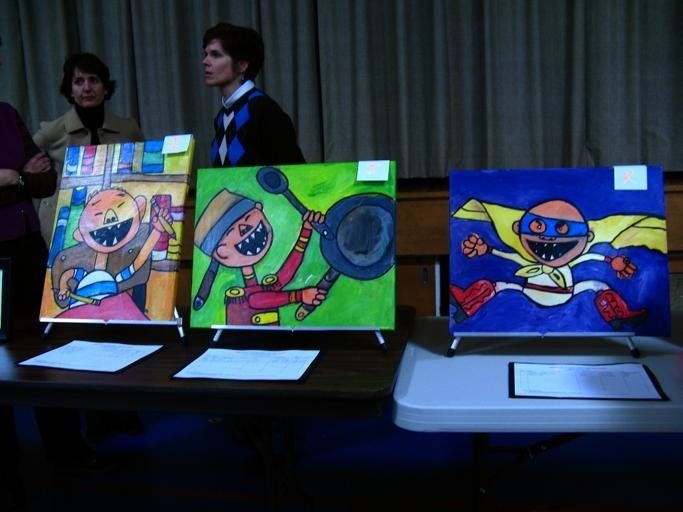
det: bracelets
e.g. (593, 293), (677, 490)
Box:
(18, 174), (25, 189)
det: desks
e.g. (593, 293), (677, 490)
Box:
(391, 317), (683, 510)
(2, 334), (401, 511)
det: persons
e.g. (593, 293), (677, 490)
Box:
(200, 22), (307, 166)
(0, 102), (64, 345)
(34, 52), (144, 248)
